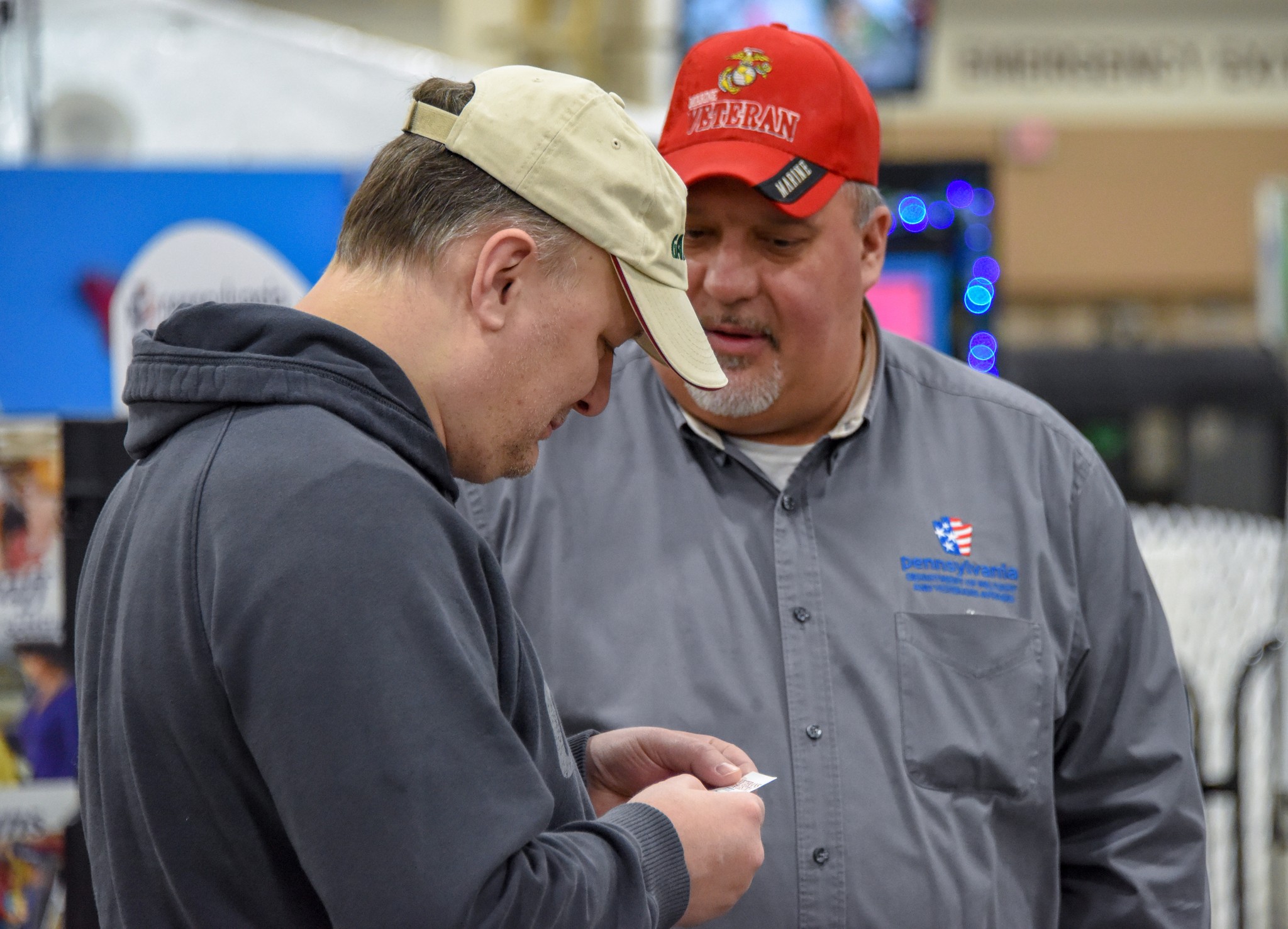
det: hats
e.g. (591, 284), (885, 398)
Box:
(402, 65), (731, 392)
(654, 23), (882, 219)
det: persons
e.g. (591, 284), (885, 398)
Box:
(10, 636), (78, 782)
(72, 61), (766, 928)
(456, 19), (1212, 929)
